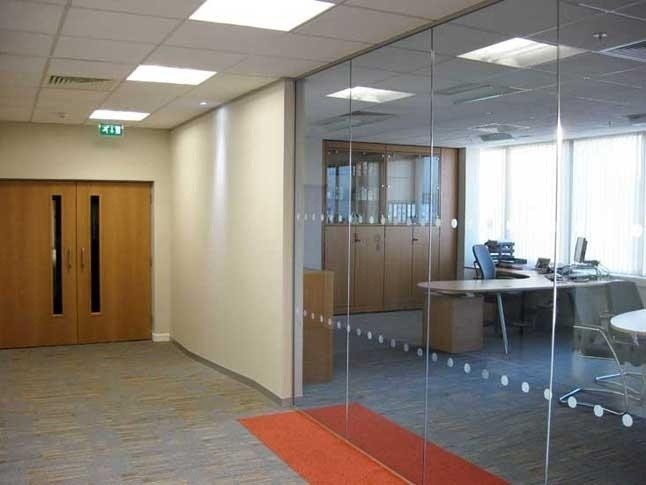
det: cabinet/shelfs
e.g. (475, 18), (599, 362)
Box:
(322, 141), (459, 321)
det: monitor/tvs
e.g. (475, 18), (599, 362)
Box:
(574, 237), (599, 267)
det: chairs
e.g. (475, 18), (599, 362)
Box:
(473, 244), (531, 336)
(559, 281), (645, 416)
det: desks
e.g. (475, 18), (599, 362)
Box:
(417, 264), (626, 355)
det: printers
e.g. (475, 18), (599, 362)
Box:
(484, 240), (515, 253)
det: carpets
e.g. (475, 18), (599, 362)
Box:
(237, 401), (511, 484)
